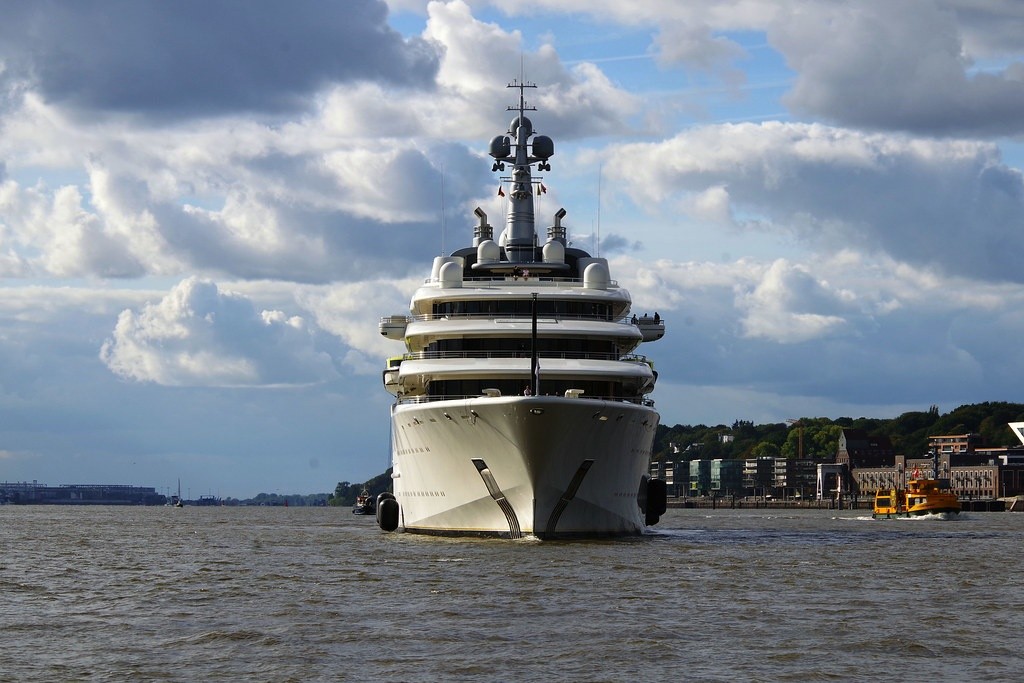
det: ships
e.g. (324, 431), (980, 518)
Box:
(378, 46), (669, 539)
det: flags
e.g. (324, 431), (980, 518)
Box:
(498, 186), (505, 197)
(537, 184), (546, 196)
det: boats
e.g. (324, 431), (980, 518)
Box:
(872, 446), (961, 517)
(352, 484), (377, 515)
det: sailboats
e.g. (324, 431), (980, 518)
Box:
(175, 477), (184, 507)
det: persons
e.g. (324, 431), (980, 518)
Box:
(524, 385), (532, 397)
(631, 312), (660, 325)
(513, 266), (530, 278)
